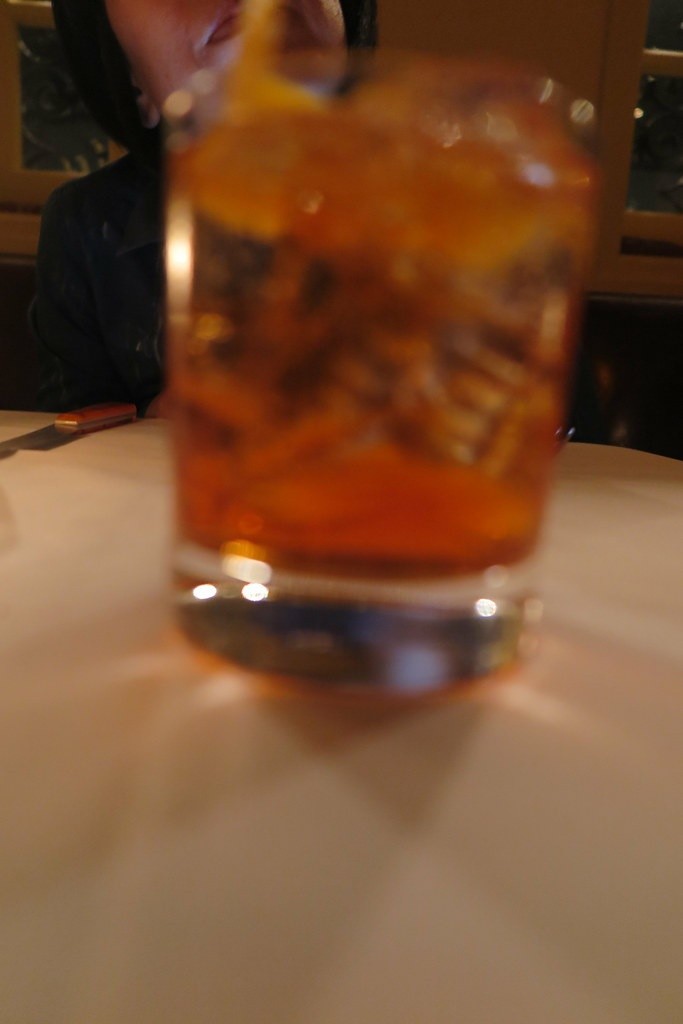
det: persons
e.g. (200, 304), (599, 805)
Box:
(22, 1), (371, 421)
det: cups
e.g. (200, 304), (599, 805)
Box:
(154, 46), (602, 695)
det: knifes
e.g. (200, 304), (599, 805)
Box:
(0, 400), (137, 459)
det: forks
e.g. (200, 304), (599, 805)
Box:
(548, 424), (576, 460)
